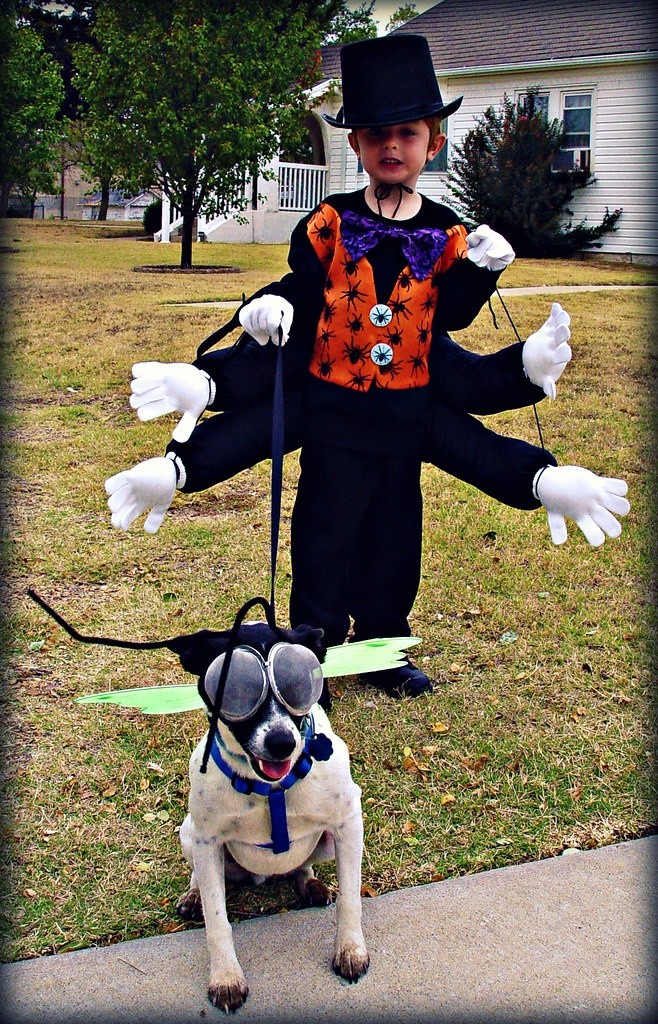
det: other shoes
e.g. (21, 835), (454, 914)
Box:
(320, 680), (331, 714)
(360, 666), (432, 699)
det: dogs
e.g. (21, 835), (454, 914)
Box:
(174, 623), (371, 1017)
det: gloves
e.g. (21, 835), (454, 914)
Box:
(465, 225), (515, 271)
(240, 294), (292, 347)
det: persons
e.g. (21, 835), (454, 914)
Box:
(236, 100), (518, 715)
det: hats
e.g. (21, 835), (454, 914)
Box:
(322, 36), (463, 129)
